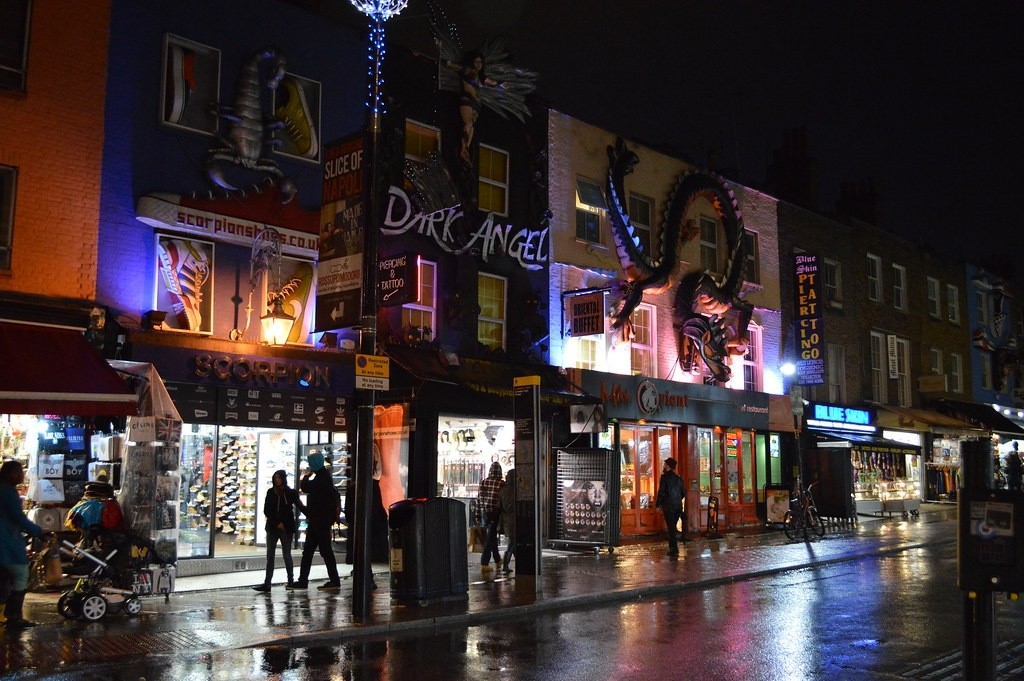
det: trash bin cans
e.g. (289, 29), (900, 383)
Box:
(388, 496), (469, 606)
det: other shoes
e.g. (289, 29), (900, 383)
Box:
(324, 580), (340, 587)
(286, 585), (293, 589)
(5, 619), (30, 629)
(667, 551), (678, 556)
(257, 584), (271, 591)
(295, 582), (307, 588)
(370, 583), (377, 589)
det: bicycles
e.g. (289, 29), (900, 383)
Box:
(782, 480), (826, 540)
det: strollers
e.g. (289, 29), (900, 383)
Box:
(47, 531), (155, 621)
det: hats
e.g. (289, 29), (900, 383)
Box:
(664, 458), (677, 468)
(90, 475), (108, 484)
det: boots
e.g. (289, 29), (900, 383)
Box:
(502, 552), (513, 572)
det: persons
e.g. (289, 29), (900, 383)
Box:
(655, 457), (687, 556)
(563, 480), (606, 540)
(476, 462), (516, 572)
(1004, 442), (1022, 490)
(0, 461), (53, 627)
(438, 425), (517, 472)
(446, 53), (512, 166)
(250, 452), (390, 591)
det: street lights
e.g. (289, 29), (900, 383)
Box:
(351, 0), (411, 612)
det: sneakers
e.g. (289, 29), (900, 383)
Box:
(495, 559), (503, 568)
(480, 565), (493, 572)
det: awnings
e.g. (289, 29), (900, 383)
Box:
(868, 399), (1024, 439)
(0, 318), (139, 416)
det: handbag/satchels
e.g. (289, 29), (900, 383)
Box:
(483, 512), (495, 521)
(496, 514), (504, 534)
